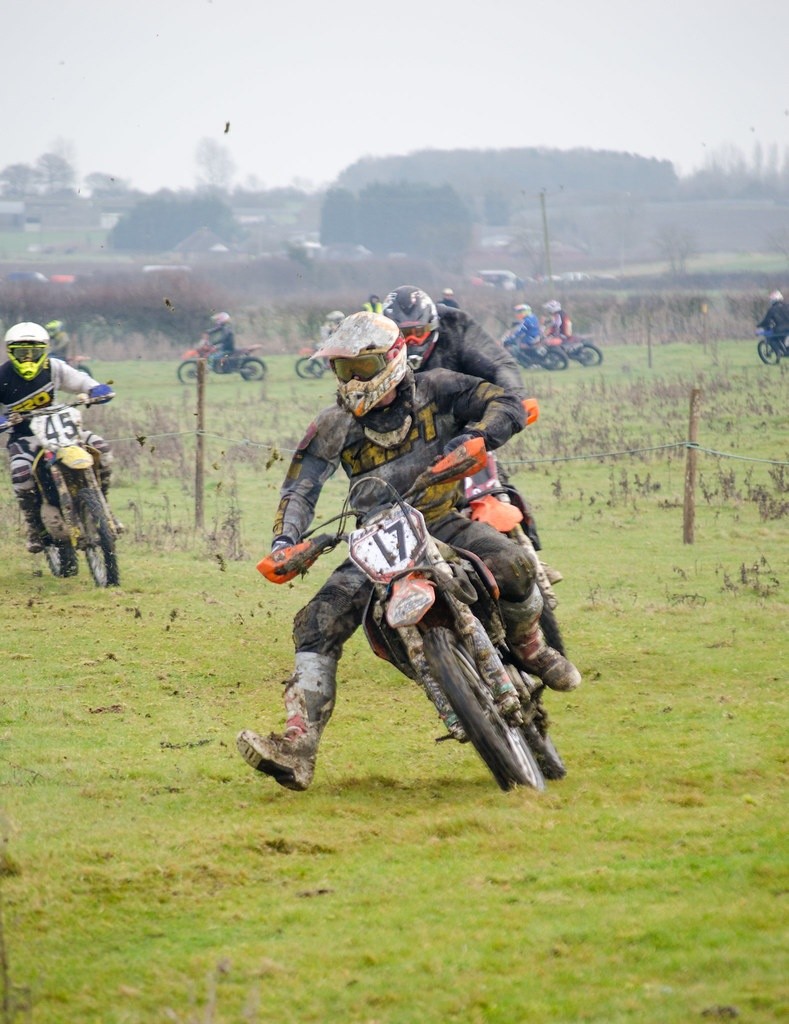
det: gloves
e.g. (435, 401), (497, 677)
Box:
(0, 415), (9, 432)
(87, 384), (116, 407)
(270, 538), (294, 553)
(444, 434), (475, 458)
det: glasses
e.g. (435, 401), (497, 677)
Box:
(400, 318), (440, 346)
(333, 354), (386, 383)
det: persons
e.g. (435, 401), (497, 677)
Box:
(316, 286), (573, 585)
(237, 312), (582, 793)
(205, 312), (236, 370)
(0, 320), (125, 555)
(755, 290), (789, 356)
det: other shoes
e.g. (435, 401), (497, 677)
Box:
(541, 560), (564, 585)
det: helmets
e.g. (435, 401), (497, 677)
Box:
(210, 312), (230, 326)
(769, 291), (782, 303)
(4, 321), (50, 381)
(45, 320), (64, 338)
(326, 311), (345, 323)
(308, 312), (406, 419)
(514, 304), (533, 320)
(383, 285), (440, 370)
(543, 299), (562, 314)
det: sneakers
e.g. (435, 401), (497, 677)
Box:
(113, 514), (123, 534)
(27, 535), (43, 554)
(503, 629), (582, 692)
(235, 731), (317, 792)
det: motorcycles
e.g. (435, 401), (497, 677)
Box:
(539, 315), (603, 367)
(754, 320), (789, 364)
(49, 353), (92, 379)
(501, 322), (568, 370)
(257, 434), (567, 794)
(177, 332), (267, 383)
(294, 325), (332, 379)
(0, 383), (126, 589)
(458, 398), (566, 660)
